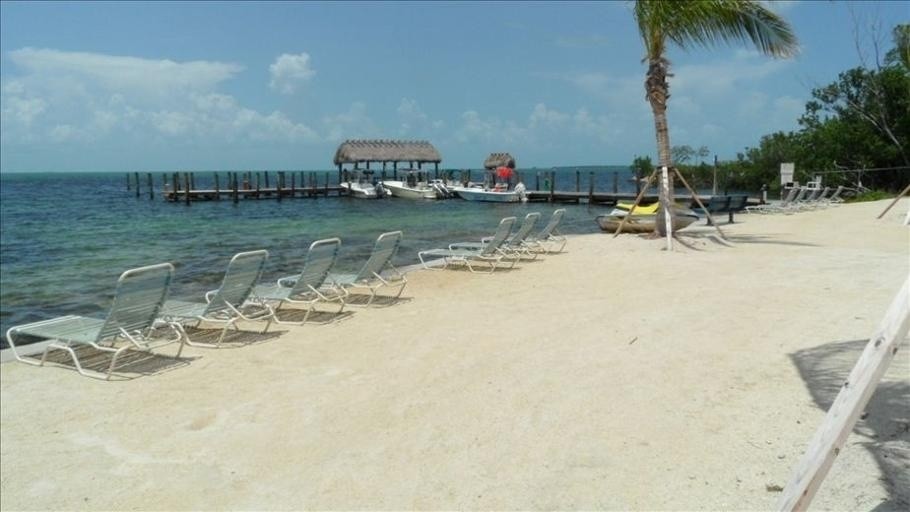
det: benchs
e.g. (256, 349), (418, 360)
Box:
(691, 194), (748, 227)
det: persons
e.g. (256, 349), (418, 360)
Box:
(407, 170), (416, 188)
(342, 167), (352, 183)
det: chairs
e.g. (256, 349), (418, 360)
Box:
(141, 248), (280, 351)
(740, 182), (845, 215)
(447, 209), (540, 267)
(416, 214), (520, 280)
(202, 236), (351, 329)
(5, 261), (190, 383)
(479, 205), (567, 254)
(274, 228), (409, 307)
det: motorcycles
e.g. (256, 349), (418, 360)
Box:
(595, 195), (699, 233)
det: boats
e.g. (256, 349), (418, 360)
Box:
(340, 166), (530, 205)
